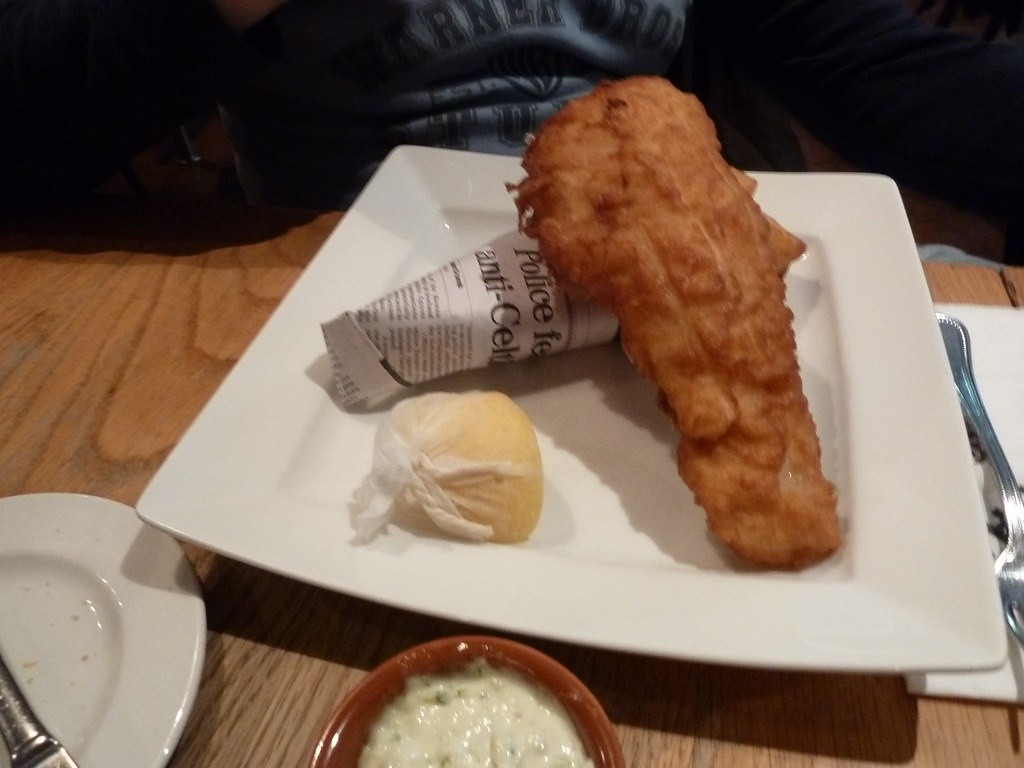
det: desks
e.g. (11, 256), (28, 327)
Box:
(0, 213), (1024, 768)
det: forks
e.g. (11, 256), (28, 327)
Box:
(938, 315), (1023, 643)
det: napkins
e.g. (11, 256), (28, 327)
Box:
(900, 303), (1024, 704)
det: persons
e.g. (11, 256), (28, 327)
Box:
(1, 0), (1024, 248)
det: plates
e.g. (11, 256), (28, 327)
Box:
(135, 145), (1009, 674)
(0, 492), (207, 768)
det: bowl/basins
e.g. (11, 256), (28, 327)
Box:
(309, 635), (627, 768)
